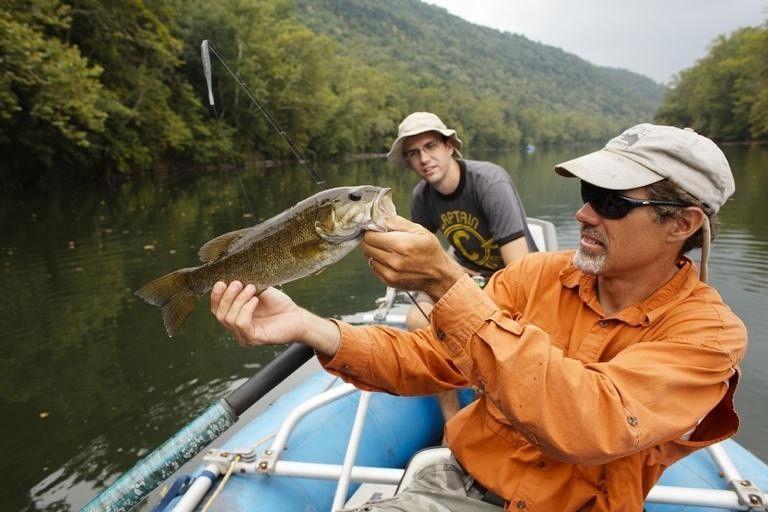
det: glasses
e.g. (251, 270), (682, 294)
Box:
(581, 178), (697, 219)
(403, 140), (445, 162)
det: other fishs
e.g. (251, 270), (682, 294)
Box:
(132, 183), (401, 338)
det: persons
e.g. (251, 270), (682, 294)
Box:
(385, 111), (542, 446)
(209, 122), (748, 512)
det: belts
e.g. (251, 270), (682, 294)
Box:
(458, 463), (508, 506)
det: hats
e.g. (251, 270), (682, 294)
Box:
(386, 112), (463, 170)
(554, 123), (735, 215)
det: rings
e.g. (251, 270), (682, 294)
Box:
(368, 257), (375, 269)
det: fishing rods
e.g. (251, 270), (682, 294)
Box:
(201, 39), (433, 323)
